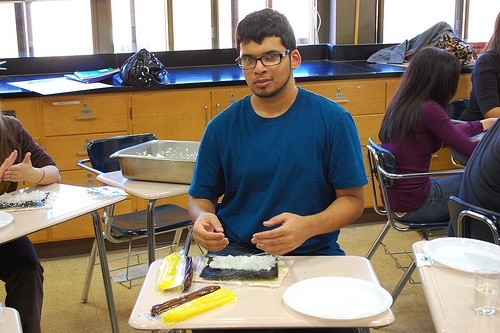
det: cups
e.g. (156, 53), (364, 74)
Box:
(472, 268), (500, 314)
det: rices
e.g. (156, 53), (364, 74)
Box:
(0, 189), (46, 206)
(136, 147), (197, 160)
(209, 254), (277, 271)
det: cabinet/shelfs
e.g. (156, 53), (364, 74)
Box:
(0, 74), (474, 262)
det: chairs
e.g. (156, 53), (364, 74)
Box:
(366, 136), (500, 303)
(77, 134), (192, 302)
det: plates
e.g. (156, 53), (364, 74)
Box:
(283, 276), (392, 320)
(422, 237), (500, 276)
(0, 210), (14, 230)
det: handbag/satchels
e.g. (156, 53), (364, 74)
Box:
(436, 34), (474, 66)
(119, 48), (168, 87)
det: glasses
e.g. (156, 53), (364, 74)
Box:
(235, 49), (291, 70)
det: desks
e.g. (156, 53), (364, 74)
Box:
(129, 255), (395, 333)
(0, 183), (129, 333)
(412, 240), (500, 333)
(95, 171), (190, 265)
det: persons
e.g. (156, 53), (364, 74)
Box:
(448, 115), (500, 244)
(378, 46), (499, 222)
(0, 112), (61, 333)
(187, 8), (370, 257)
(451, 11), (500, 167)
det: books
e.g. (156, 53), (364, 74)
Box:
(65, 67), (121, 84)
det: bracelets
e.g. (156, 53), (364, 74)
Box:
(36, 167), (45, 185)
